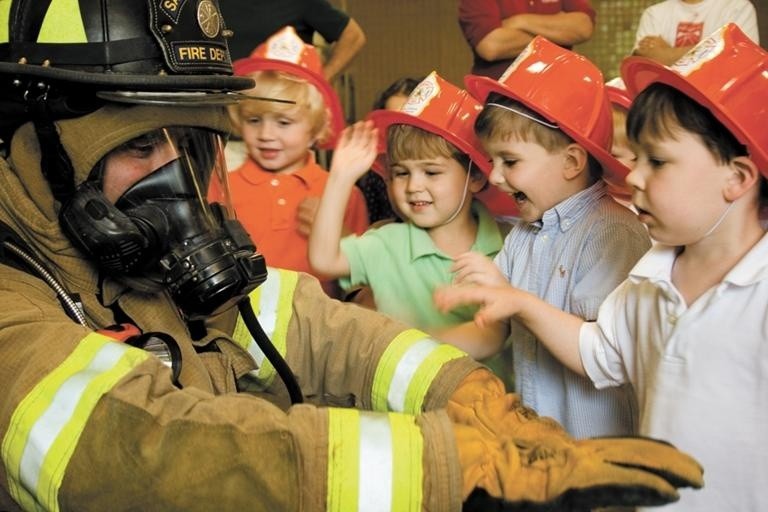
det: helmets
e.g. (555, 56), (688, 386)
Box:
(365, 68), (492, 181)
(621, 22), (766, 174)
(462, 34), (631, 188)
(0, 0), (255, 123)
(225, 26), (344, 150)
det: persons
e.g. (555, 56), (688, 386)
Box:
(357, 79), (421, 229)
(630, 0), (761, 67)
(0, 0), (705, 511)
(457, 2), (595, 82)
(212, 0), (365, 174)
(602, 74), (658, 248)
(412, 32), (655, 440)
(428, 26), (768, 511)
(303, 70), (514, 398)
(193, 24), (370, 305)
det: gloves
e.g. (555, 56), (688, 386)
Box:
(443, 366), (705, 509)
